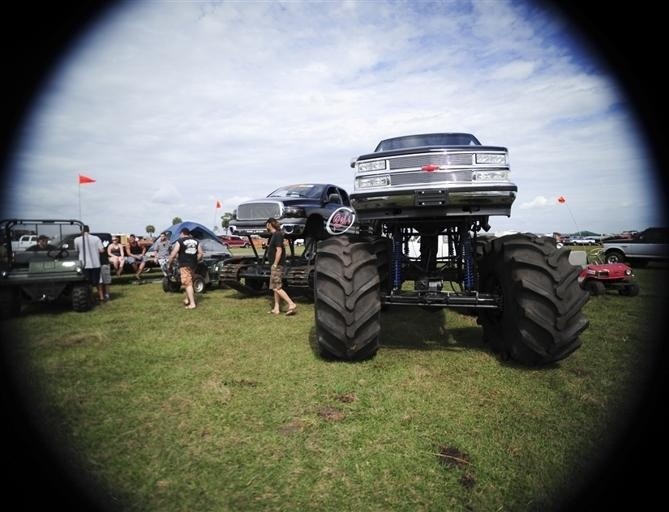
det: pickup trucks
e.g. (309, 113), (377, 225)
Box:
(11, 234), (52, 252)
(51, 232), (164, 280)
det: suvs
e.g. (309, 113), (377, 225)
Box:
(602, 227), (669, 264)
(0, 219), (91, 319)
(306, 133), (590, 365)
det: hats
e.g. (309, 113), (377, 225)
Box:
(266, 218), (276, 223)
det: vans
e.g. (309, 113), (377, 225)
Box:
(57, 232), (110, 250)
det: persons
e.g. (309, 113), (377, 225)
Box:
(266, 217), (298, 315)
(74, 224), (203, 310)
(25, 235), (59, 250)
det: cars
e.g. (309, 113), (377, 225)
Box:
(162, 237), (233, 292)
(555, 233), (601, 244)
(577, 248), (639, 297)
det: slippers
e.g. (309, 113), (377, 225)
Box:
(267, 305), (298, 315)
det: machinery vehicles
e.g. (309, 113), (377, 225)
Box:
(221, 183), (354, 302)
